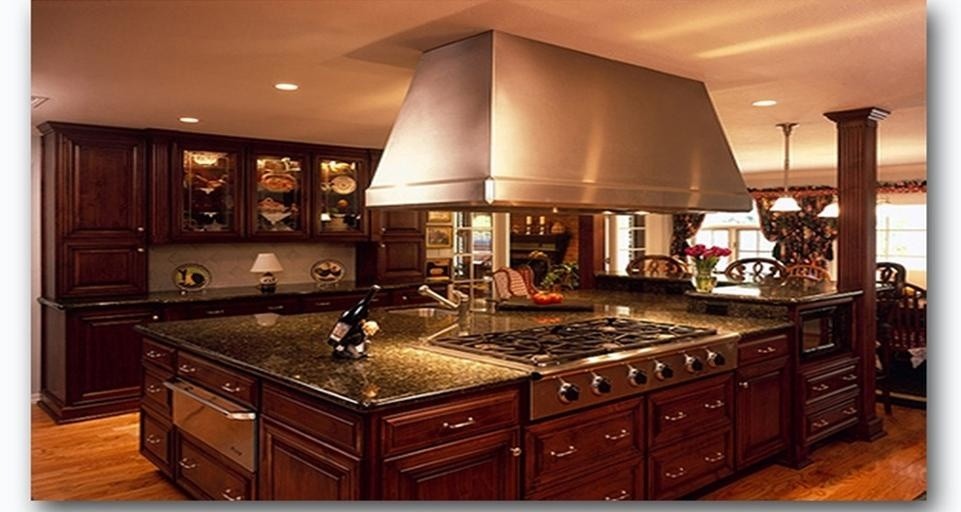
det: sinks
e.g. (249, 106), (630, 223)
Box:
(387, 303), (466, 317)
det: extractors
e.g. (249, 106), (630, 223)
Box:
(362, 28), (752, 211)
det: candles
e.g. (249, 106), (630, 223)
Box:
(540, 215), (546, 226)
(526, 215), (533, 226)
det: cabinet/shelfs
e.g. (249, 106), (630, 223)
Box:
(247, 137), (314, 239)
(735, 332), (793, 468)
(260, 374), (365, 500)
(454, 212), (497, 296)
(363, 381), (524, 501)
(136, 323), (175, 484)
(425, 224), (453, 249)
(154, 126), (246, 243)
(521, 397), (646, 500)
(36, 121), (151, 300)
(309, 143), (372, 243)
(648, 371), (735, 500)
(174, 346), (258, 503)
(371, 149), (428, 286)
(791, 296), (867, 468)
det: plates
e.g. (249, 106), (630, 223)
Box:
(310, 259), (347, 282)
(263, 174), (298, 192)
(331, 174), (357, 194)
(171, 264), (211, 292)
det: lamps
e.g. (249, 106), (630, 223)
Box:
(769, 122), (806, 213)
(252, 254), (282, 292)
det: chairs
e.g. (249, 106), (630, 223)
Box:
(885, 283), (926, 416)
(623, 250), (908, 287)
(513, 262), (541, 295)
(817, 162), (841, 218)
(494, 266), (531, 300)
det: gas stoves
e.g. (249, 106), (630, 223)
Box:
(429, 316), (743, 422)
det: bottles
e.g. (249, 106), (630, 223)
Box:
(327, 284), (379, 347)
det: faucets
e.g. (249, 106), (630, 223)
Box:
(418, 284), (457, 310)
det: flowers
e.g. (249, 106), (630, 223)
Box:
(684, 242), (733, 269)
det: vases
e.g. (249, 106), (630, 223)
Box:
(691, 264), (718, 292)
